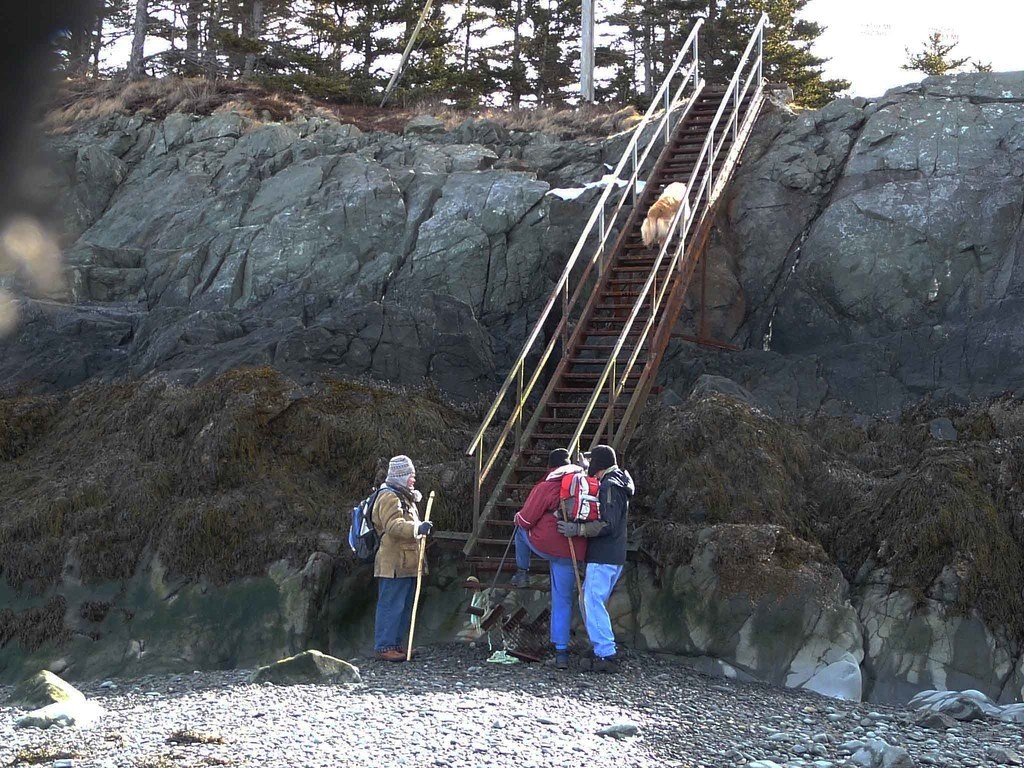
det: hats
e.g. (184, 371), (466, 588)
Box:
(588, 445), (616, 477)
(385, 455), (422, 502)
(549, 448), (569, 468)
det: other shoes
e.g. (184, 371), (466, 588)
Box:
(487, 651), (507, 663)
(511, 569), (530, 587)
(502, 655), (519, 665)
(556, 654), (569, 669)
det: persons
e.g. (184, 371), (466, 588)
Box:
(510, 448), (586, 669)
(556, 446), (634, 675)
(372, 455), (433, 661)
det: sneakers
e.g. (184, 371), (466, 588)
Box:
(580, 657), (616, 673)
(579, 650), (619, 659)
(374, 650), (406, 661)
(399, 648), (416, 654)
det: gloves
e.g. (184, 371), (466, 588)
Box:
(418, 522), (431, 535)
(557, 521), (586, 537)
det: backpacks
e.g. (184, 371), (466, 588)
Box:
(349, 487), (406, 558)
(560, 473), (600, 523)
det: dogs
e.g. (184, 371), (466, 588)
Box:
(640, 181), (691, 254)
